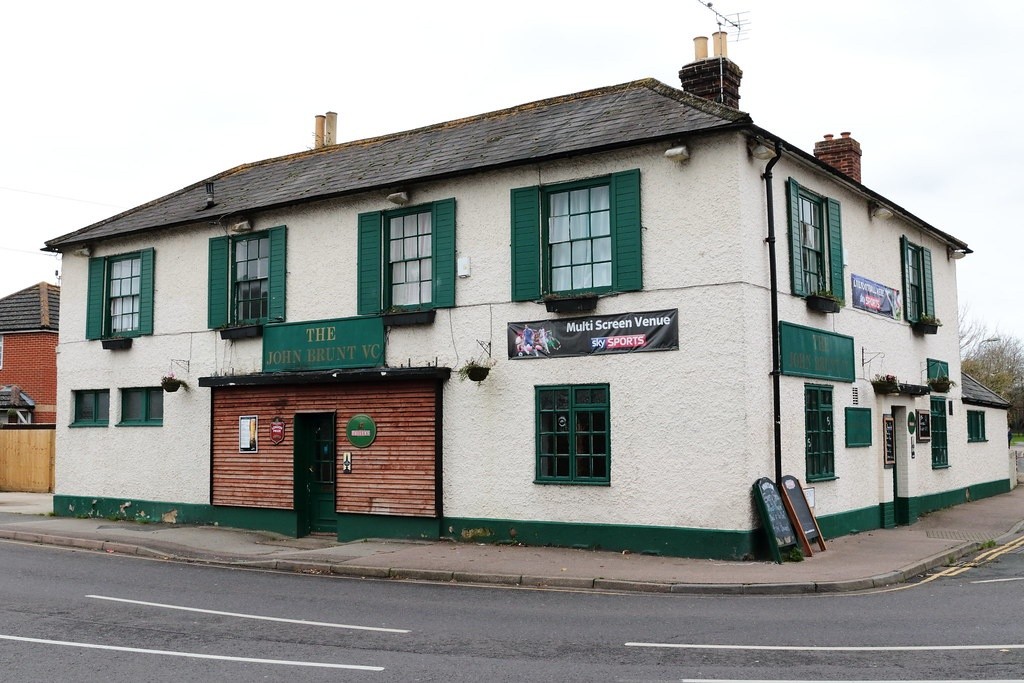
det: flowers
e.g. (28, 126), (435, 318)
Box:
(870, 372), (900, 388)
(159, 371), (191, 392)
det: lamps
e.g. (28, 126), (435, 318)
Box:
(663, 145), (690, 164)
(73, 246), (93, 257)
(231, 218), (252, 233)
(386, 190), (411, 205)
(749, 137), (776, 161)
(869, 200), (894, 221)
(947, 242), (965, 261)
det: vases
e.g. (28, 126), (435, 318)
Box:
(872, 380), (898, 394)
(163, 383), (182, 393)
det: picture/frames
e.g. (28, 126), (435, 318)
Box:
(778, 320), (856, 383)
(925, 359), (950, 392)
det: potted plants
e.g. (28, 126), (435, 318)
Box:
(543, 292), (598, 314)
(805, 291), (845, 314)
(378, 304), (437, 328)
(100, 334), (133, 350)
(456, 358), (493, 385)
(926, 374), (956, 393)
(907, 311), (940, 336)
(214, 321), (264, 341)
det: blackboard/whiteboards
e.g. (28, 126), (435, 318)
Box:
(752, 477), (798, 550)
(780, 475), (821, 540)
